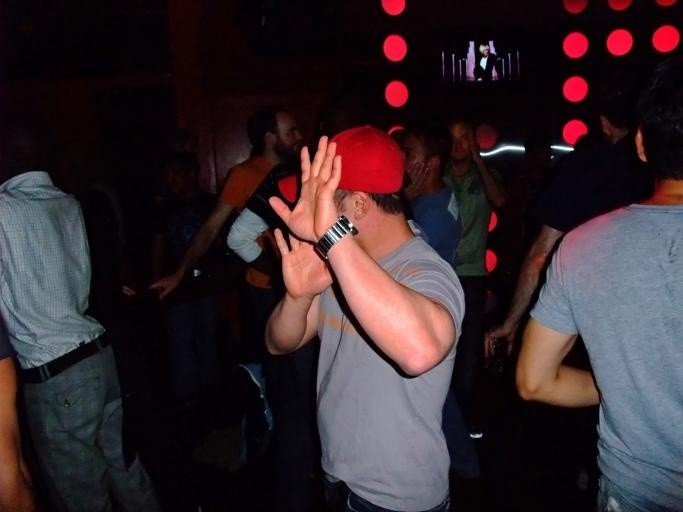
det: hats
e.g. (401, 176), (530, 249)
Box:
(324, 125), (405, 194)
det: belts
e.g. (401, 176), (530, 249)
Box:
(21, 328), (114, 385)
